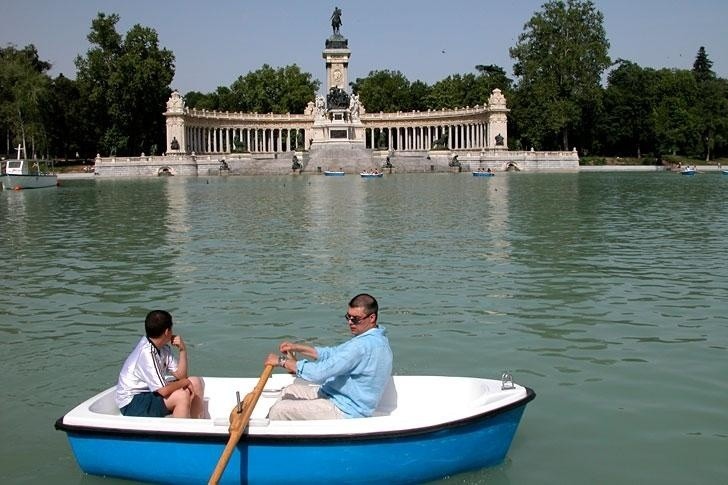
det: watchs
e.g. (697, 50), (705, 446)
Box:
(278, 354), (287, 367)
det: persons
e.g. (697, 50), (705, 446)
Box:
(432, 130), (450, 150)
(386, 157), (392, 168)
(32, 162), (38, 175)
(329, 6), (343, 26)
(263, 293), (395, 420)
(673, 160), (697, 171)
(114, 309), (205, 418)
(218, 159), (229, 170)
(80, 165), (95, 173)
(170, 136), (180, 149)
(361, 168), (381, 174)
(477, 167), (496, 173)
(328, 85), (350, 109)
(450, 154), (459, 167)
(292, 156), (301, 169)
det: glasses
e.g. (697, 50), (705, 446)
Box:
(344, 308), (371, 324)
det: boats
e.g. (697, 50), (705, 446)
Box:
(55, 372), (535, 485)
(360, 172), (383, 177)
(472, 172), (494, 176)
(680, 170), (696, 176)
(0, 159), (58, 189)
(324, 171), (344, 176)
